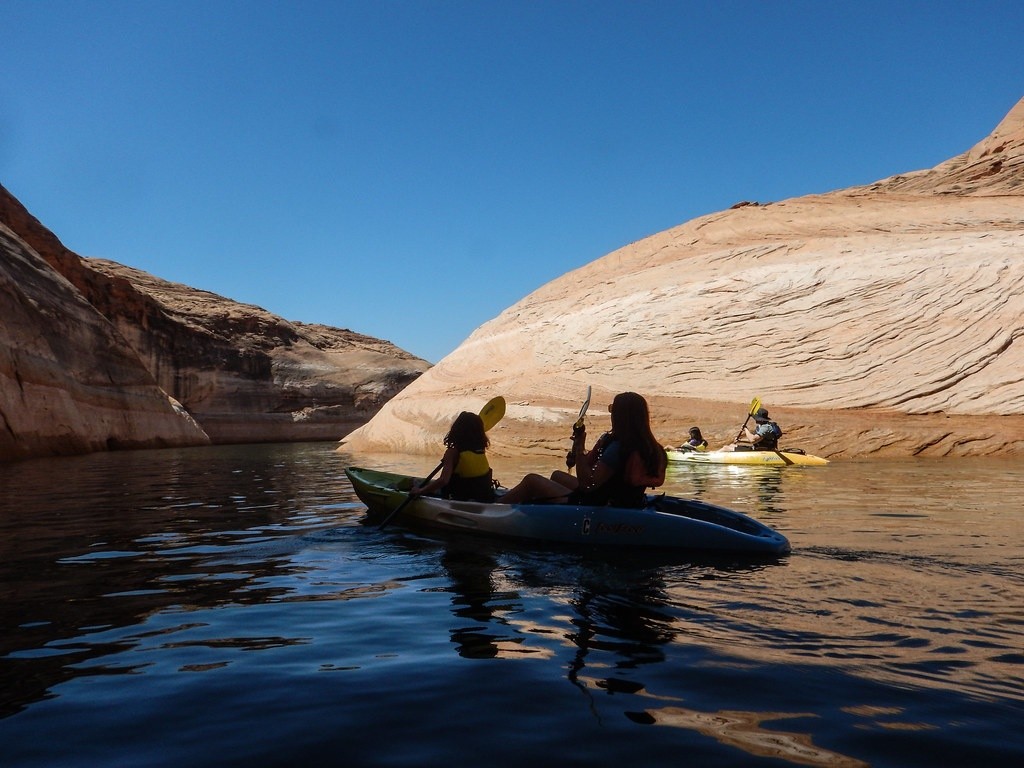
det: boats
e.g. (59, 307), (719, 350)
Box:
(665, 450), (832, 467)
(344, 465), (792, 556)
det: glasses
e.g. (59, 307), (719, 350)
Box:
(608, 404), (614, 414)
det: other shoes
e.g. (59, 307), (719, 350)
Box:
(412, 477), (422, 488)
(389, 484), (398, 491)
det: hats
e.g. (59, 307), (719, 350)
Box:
(752, 408), (772, 421)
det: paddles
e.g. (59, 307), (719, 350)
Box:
(567, 385), (591, 475)
(377, 396), (506, 531)
(736, 396), (761, 440)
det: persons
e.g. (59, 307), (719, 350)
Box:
(387, 411), (491, 503)
(663, 427), (703, 452)
(709, 407), (777, 452)
(486, 391), (668, 510)
